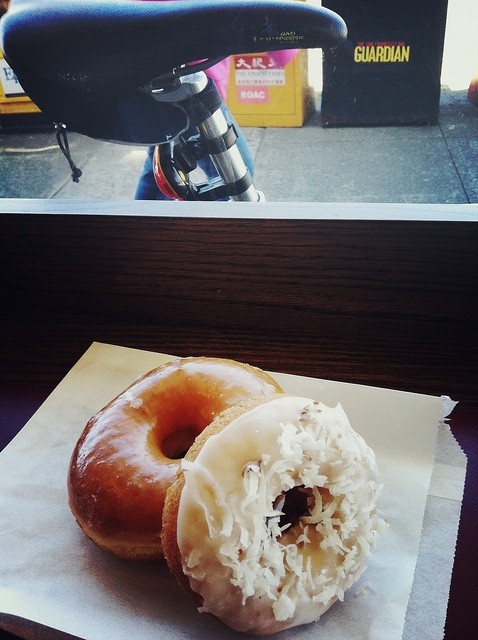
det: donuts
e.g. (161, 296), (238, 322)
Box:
(67, 357), (285, 563)
(160, 395), (391, 636)
